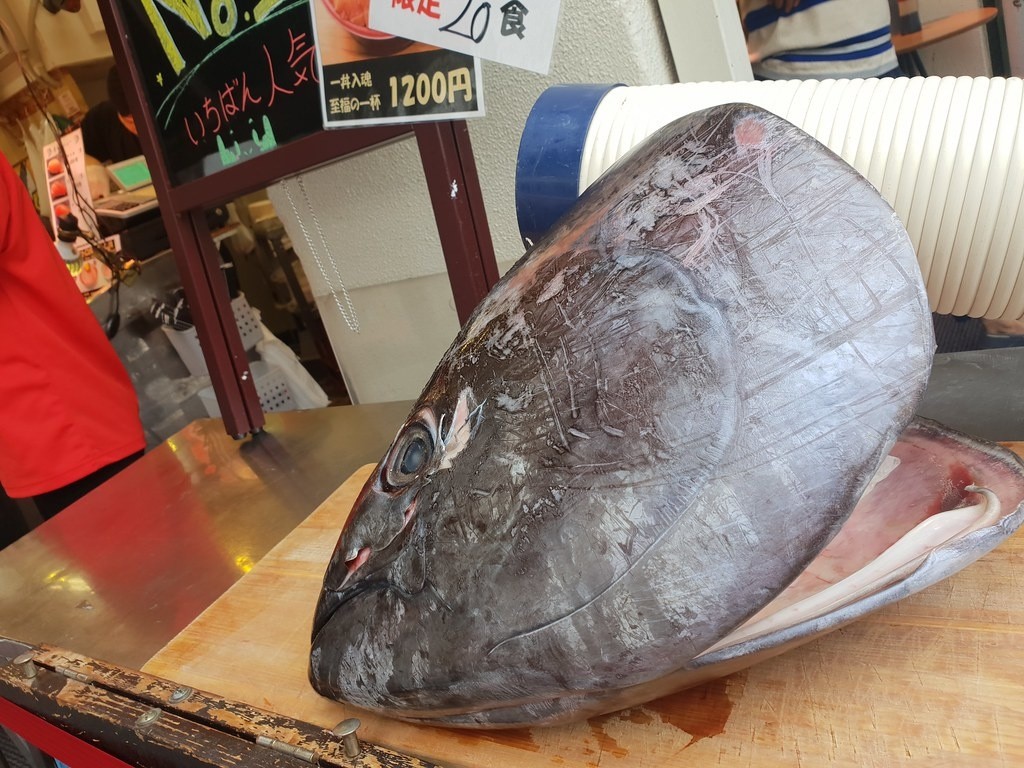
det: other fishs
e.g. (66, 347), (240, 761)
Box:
(306, 101), (1024, 730)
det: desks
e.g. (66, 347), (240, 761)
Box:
(0, 346), (1024, 768)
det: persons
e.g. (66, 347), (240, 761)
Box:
(1, 150), (148, 519)
(82, 66), (228, 256)
(738, 1), (904, 81)
